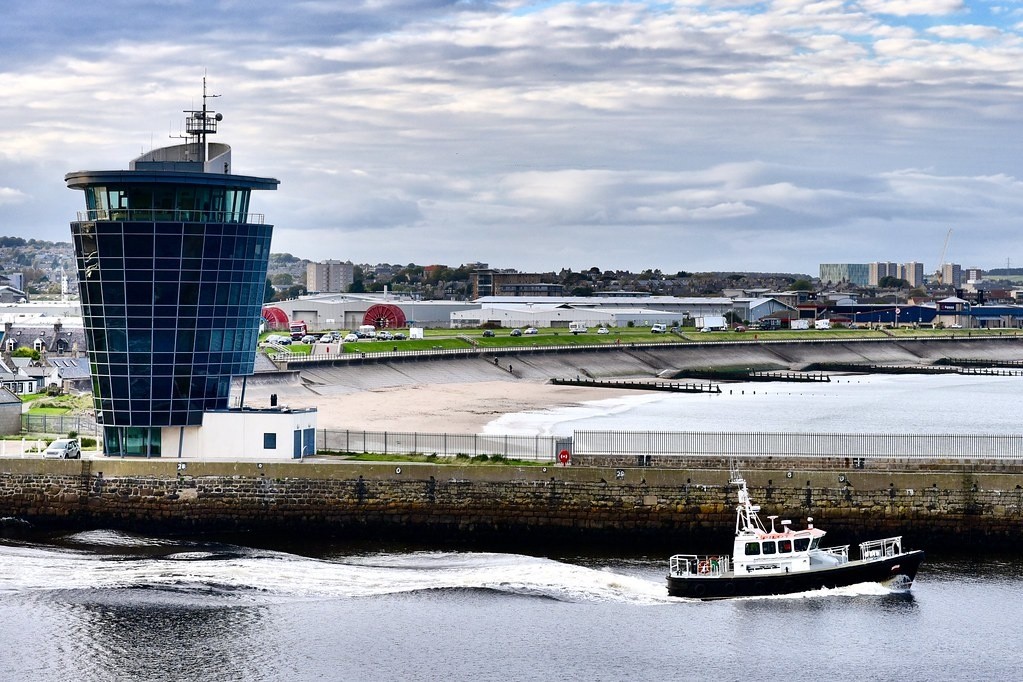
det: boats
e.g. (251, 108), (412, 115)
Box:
(665, 456), (927, 602)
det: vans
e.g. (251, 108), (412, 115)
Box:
(650, 323), (667, 334)
(356, 324), (375, 339)
(568, 321), (588, 334)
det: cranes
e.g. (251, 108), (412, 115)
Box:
(933, 228), (953, 285)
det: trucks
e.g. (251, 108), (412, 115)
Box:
(694, 316), (729, 331)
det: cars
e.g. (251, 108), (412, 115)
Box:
(265, 319), (341, 346)
(598, 328), (609, 334)
(510, 328), (522, 336)
(483, 330), (496, 338)
(670, 327), (682, 333)
(700, 327), (712, 333)
(950, 323), (963, 330)
(42, 439), (81, 460)
(394, 333), (408, 341)
(524, 328), (539, 335)
(734, 326), (745, 332)
(344, 333), (359, 343)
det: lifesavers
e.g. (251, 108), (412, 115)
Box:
(698, 561), (710, 574)
(708, 557), (719, 563)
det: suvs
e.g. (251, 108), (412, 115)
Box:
(376, 331), (394, 341)
